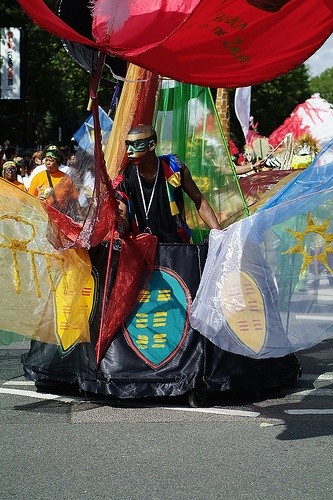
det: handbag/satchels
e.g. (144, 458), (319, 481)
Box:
(60, 206), (81, 224)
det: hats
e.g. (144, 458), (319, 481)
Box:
(2, 161), (17, 178)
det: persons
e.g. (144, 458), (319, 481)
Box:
(0, 144), (95, 212)
(183, 135), (266, 230)
(111, 123), (222, 244)
(28, 149), (84, 219)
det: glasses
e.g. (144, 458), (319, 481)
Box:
(5, 168), (16, 172)
